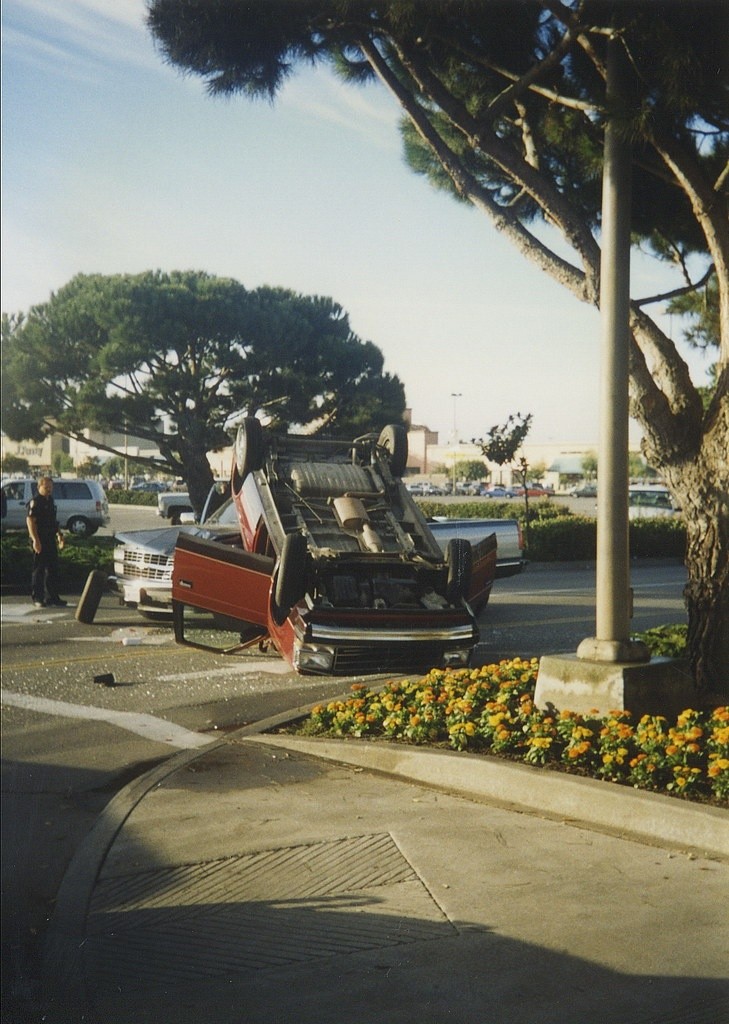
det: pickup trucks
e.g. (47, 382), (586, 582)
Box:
(110, 487), (528, 628)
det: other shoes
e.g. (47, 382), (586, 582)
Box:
(32, 597), (45, 607)
(49, 599), (67, 606)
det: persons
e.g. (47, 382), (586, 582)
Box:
(25, 477), (69, 607)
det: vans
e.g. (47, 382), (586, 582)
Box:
(0, 479), (111, 537)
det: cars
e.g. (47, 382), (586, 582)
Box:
(155, 477), (232, 526)
(105, 474), (180, 492)
(405, 481), (545, 496)
(570, 485), (596, 498)
(628, 484), (683, 521)
(515, 487), (556, 498)
(480, 488), (517, 499)
(171, 411), (498, 675)
